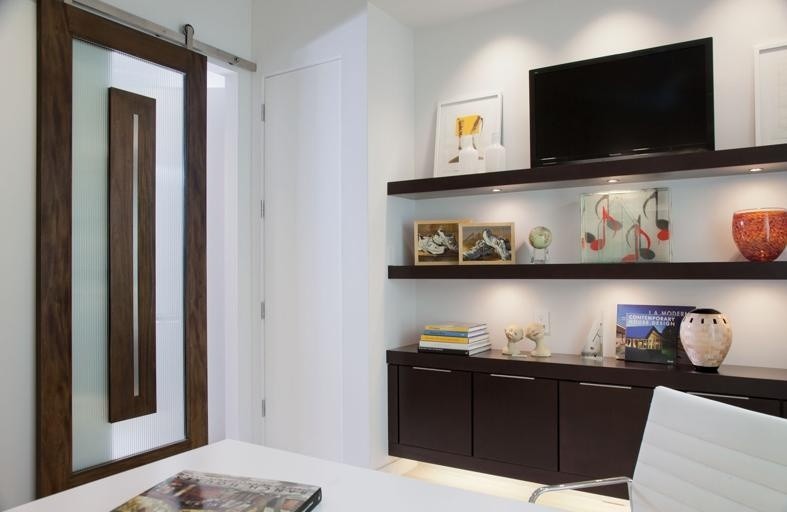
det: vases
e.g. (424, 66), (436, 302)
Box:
(733, 210), (787, 262)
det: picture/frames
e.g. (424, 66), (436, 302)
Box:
(414, 221), (470, 266)
(432, 92), (502, 179)
(754, 38), (787, 148)
(460, 221), (517, 266)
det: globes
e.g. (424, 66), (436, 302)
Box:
(529, 225), (553, 264)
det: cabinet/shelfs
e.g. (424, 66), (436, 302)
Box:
(386, 343), (787, 500)
(388, 144), (787, 279)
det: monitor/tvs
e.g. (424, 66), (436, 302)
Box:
(527, 38), (714, 168)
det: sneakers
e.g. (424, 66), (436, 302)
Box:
(433, 230), (458, 250)
(463, 239), (494, 260)
(483, 228), (512, 260)
(418, 234), (447, 255)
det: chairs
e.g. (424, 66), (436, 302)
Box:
(528, 386), (787, 512)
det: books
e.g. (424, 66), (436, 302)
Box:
(615, 304), (696, 367)
(415, 317), (491, 358)
(112, 467), (323, 511)
(626, 312), (678, 366)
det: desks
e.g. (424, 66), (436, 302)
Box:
(0, 441), (573, 512)
